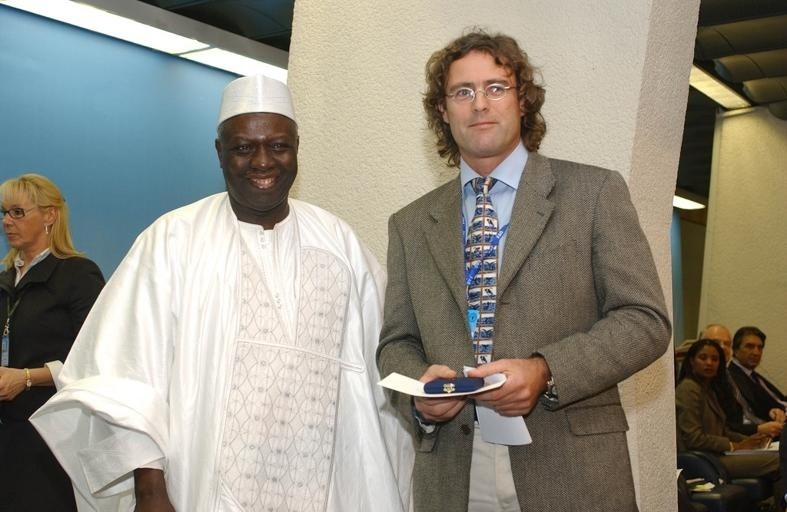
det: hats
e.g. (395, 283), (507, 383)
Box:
(217, 76), (297, 126)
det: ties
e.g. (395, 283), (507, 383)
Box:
(466, 177), (498, 367)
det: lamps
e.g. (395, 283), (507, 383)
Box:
(673, 188), (707, 211)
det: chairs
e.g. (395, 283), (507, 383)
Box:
(684, 449), (773, 501)
(678, 453), (764, 512)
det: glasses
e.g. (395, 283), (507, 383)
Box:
(444, 84), (515, 104)
(0, 208), (35, 219)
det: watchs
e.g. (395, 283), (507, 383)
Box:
(544, 374), (557, 398)
(24, 367), (32, 392)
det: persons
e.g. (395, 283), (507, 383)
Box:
(729, 326), (787, 421)
(700, 324), (787, 439)
(676, 339), (787, 512)
(28, 76), (416, 512)
(0, 174), (105, 512)
(377, 35), (670, 512)
